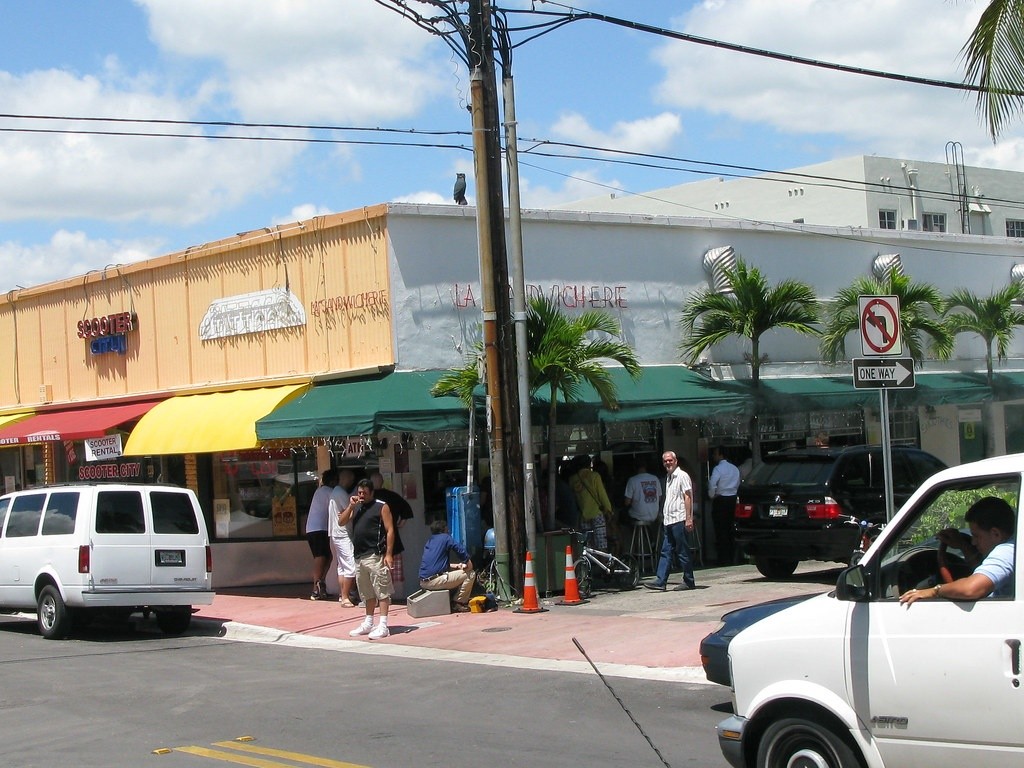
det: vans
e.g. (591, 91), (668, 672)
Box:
(698, 452), (1024, 768)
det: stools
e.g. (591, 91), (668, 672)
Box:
(630, 520), (665, 576)
(687, 513), (705, 569)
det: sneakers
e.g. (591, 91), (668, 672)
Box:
(310, 591), (320, 600)
(644, 582), (666, 590)
(673, 582), (691, 590)
(349, 622), (375, 636)
(369, 626), (390, 639)
(316, 580), (328, 600)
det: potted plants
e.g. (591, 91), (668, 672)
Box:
(430, 291), (642, 593)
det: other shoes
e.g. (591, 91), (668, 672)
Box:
(451, 601), (470, 612)
(358, 597), (391, 607)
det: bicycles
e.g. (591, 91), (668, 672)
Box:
(560, 523), (640, 599)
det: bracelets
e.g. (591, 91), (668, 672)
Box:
(460, 563), (462, 567)
(934, 584), (942, 598)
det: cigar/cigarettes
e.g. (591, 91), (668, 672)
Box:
(388, 568), (394, 570)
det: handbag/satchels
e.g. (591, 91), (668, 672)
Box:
(377, 535), (387, 555)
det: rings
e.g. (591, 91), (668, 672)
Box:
(690, 526), (691, 528)
(913, 589), (916, 592)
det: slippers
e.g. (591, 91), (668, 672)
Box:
(339, 595), (356, 602)
(342, 602), (355, 608)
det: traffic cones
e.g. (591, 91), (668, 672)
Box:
(511, 551), (550, 614)
(554, 545), (591, 607)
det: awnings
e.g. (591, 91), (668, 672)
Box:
(123, 383), (316, 455)
(0, 401), (160, 448)
(255, 364), (1024, 440)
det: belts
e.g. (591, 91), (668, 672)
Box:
(419, 572), (443, 582)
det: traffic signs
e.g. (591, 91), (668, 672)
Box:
(851, 357), (916, 390)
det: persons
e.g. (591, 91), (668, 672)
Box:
(327, 470), (356, 608)
(338, 479), (395, 640)
(617, 454), (662, 525)
(737, 448), (753, 478)
(357, 474), (413, 608)
(898, 496), (1016, 606)
(418, 521), (476, 614)
(707, 445), (740, 565)
(306, 469), (339, 600)
(538, 454), (616, 577)
(644, 450), (696, 590)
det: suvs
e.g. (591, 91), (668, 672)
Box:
(212, 470), (321, 540)
(732, 443), (950, 581)
(0, 481), (216, 640)
